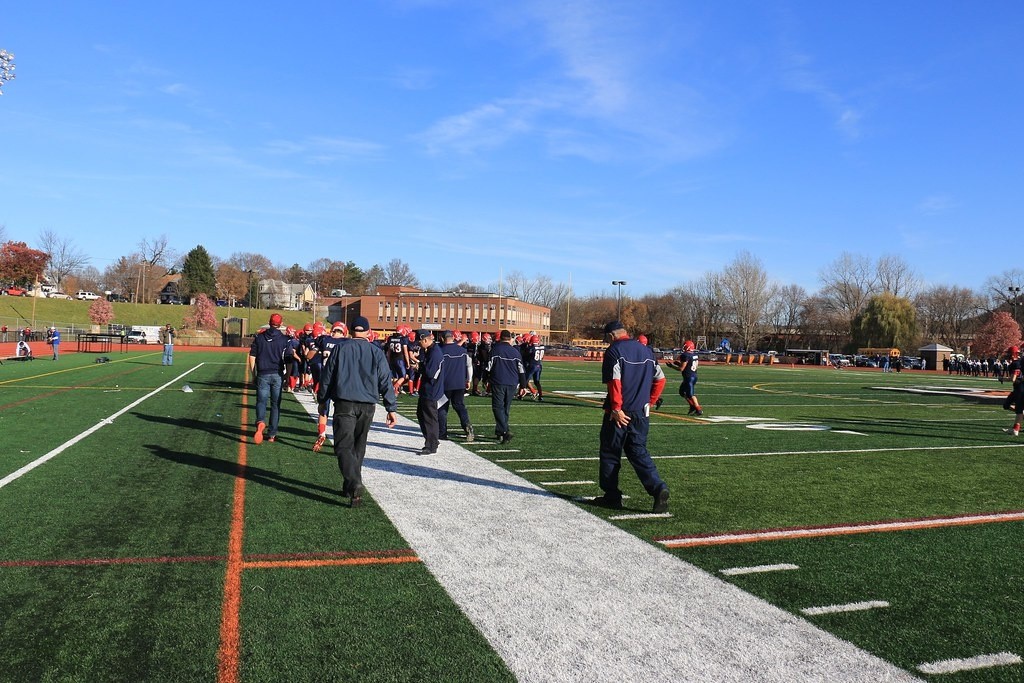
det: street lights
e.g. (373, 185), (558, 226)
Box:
(1009, 287), (1021, 321)
(612, 281), (627, 322)
(711, 303), (721, 350)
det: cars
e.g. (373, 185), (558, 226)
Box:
(107, 294), (130, 303)
(166, 298), (183, 304)
(260, 324), (295, 331)
(545, 344), (588, 357)
(46, 292), (71, 300)
(654, 347), (778, 356)
(829, 354), (922, 361)
(122, 331), (147, 344)
(1, 285), (29, 297)
(216, 300), (228, 307)
(235, 300), (255, 309)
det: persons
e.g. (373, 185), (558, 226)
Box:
(482, 329), (527, 443)
(249, 314), (293, 444)
(851, 354), (857, 367)
(947, 357), (1012, 378)
(16, 340), (30, 360)
(2, 325), (8, 342)
(1003, 343), (1024, 436)
(921, 358), (926, 370)
(48, 327), (60, 361)
(875, 353), (903, 374)
(666, 340), (703, 416)
(258, 322), (546, 402)
(312, 316), (397, 508)
(411, 329), (445, 455)
(636, 335), (663, 410)
(593, 321), (669, 513)
(439, 330), (474, 442)
(120, 328), (125, 343)
(162, 324), (176, 366)
(25, 327), (31, 342)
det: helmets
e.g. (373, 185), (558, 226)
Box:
(257, 321), (540, 345)
(1008, 346), (1020, 357)
(684, 341), (694, 351)
(637, 335), (647, 345)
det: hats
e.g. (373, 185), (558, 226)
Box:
(603, 321), (625, 343)
(442, 330), (452, 338)
(270, 314), (282, 325)
(500, 330), (511, 340)
(350, 316), (369, 332)
(413, 329), (430, 344)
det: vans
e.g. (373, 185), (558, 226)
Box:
(74, 292), (101, 300)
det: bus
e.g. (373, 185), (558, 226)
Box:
(858, 348), (900, 357)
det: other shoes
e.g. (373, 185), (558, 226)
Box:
(688, 404), (703, 416)
(501, 432), (514, 445)
(1002, 428), (1018, 436)
(497, 435), (503, 440)
(655, 398), (663, 410)
(281, 382), (543, 402)
(422, 446), (436, 454)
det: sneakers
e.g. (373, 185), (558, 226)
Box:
(254, 421), (265, 444)
(654, 488), (669, 513)
(594, 496), (622, 507)
(465, 424), (474, 441)
(351, 483), (365, 508)
(313, 434), (325, 452)
(268, 436), (276, 442)
